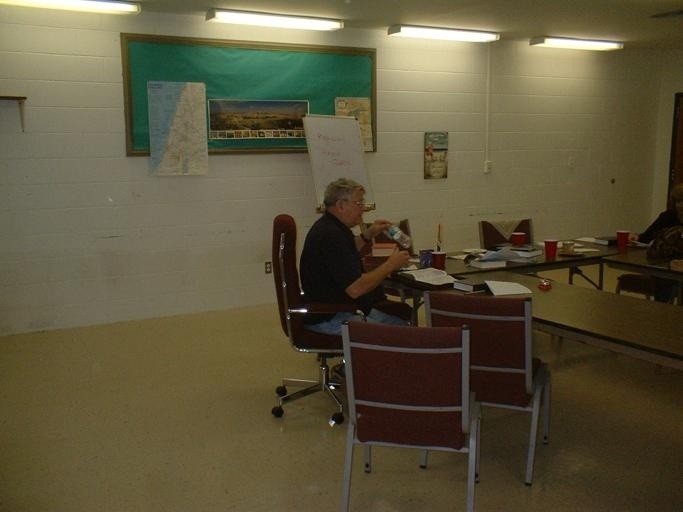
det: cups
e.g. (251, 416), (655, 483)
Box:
(511, 232), (526, 247)
(544, 240), (558, 262)
(432, 251), (446, 269)
(617, 231), (630, 242)
(562, 242), (575, 253)
(418, 248), (434, 267)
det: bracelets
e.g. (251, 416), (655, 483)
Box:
(359, 232), (372, 244)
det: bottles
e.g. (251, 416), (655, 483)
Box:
(384, 224), (413, 251)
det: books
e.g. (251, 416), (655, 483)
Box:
(468, 251), (506, 270)
(453, 277), (488, 293)
(592, 235), (618, 247)
(496, 243), (544, 259)
(396, 266), (457, 286)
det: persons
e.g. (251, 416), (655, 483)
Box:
(624, 181), (682, 304)
(300, 174), (412, 397)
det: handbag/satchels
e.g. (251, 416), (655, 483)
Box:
(646, 226), (682, 258)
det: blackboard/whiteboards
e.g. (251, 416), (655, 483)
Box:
(123, 34), (378, 157)
(301, 113), (378, 212)
